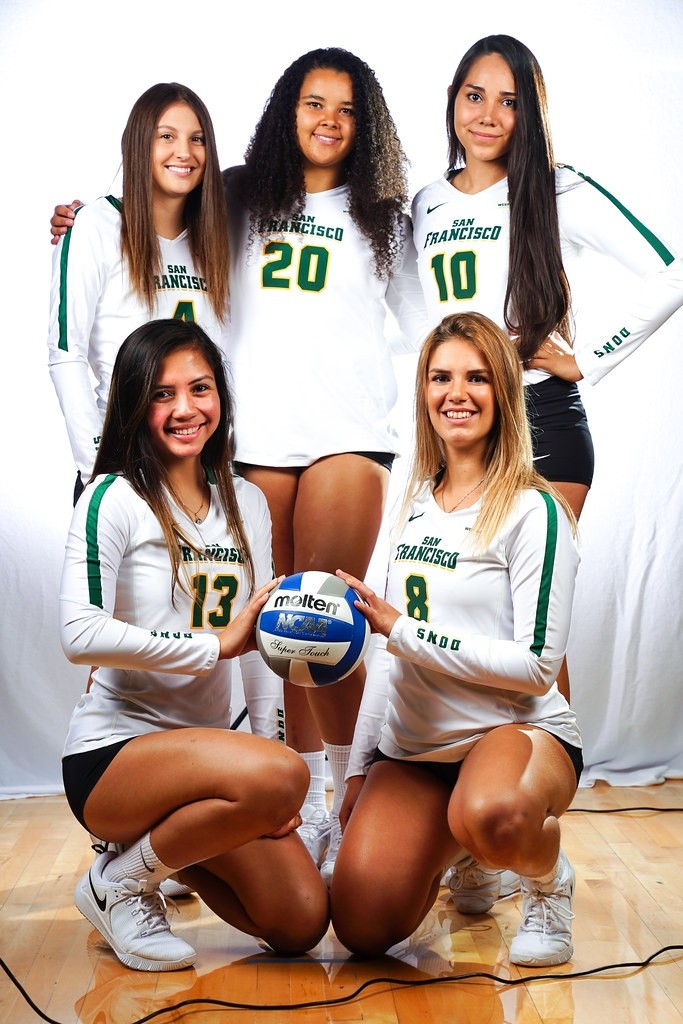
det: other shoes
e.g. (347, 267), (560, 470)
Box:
(439, 867), (520, 897)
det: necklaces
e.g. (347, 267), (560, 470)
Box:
(165, 466), (206, 523)
(439, 475), (487, 513)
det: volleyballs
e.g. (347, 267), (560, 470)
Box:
(251, 571), (374, 691)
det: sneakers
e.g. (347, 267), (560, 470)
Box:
(295, 803), (329, 868)
(72, 844), (197, 971)
(90, 833), (195, 896)
(320, 809), (343, 889)
(509, 848), (576, 967)
(448, 856), (509, 915)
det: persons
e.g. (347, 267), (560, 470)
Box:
(329, 311), (587, 971)
(49, 46), (418, 882)
(408, 36), (683, 914)
(46, 81), (233, 896)
(61, 318), (330, 974)
